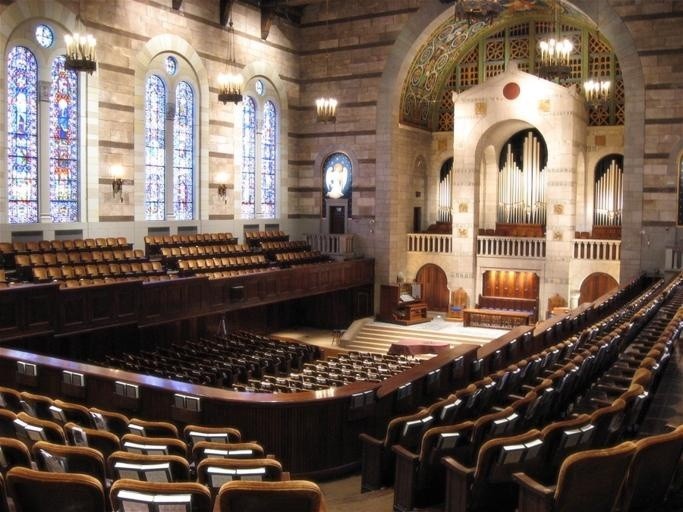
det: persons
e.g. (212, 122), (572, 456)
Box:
(325, 163), (345, 199)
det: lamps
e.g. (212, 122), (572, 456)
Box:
(217, 1), (243, 106)
(64, 0), (96, 76)
(581, 1), (611, 104)
(108, 163), (125, 202)
(216, 172), (229, 206)
(315, 96), (337, 124)
(538, 1), (572, 76)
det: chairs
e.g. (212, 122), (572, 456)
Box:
(0, 229), (327, 291)
(358, 271), (682, 511)
(423, 224), (621, 244)
(0, 391), (322, 511)
(83, 329), (359, 394)
